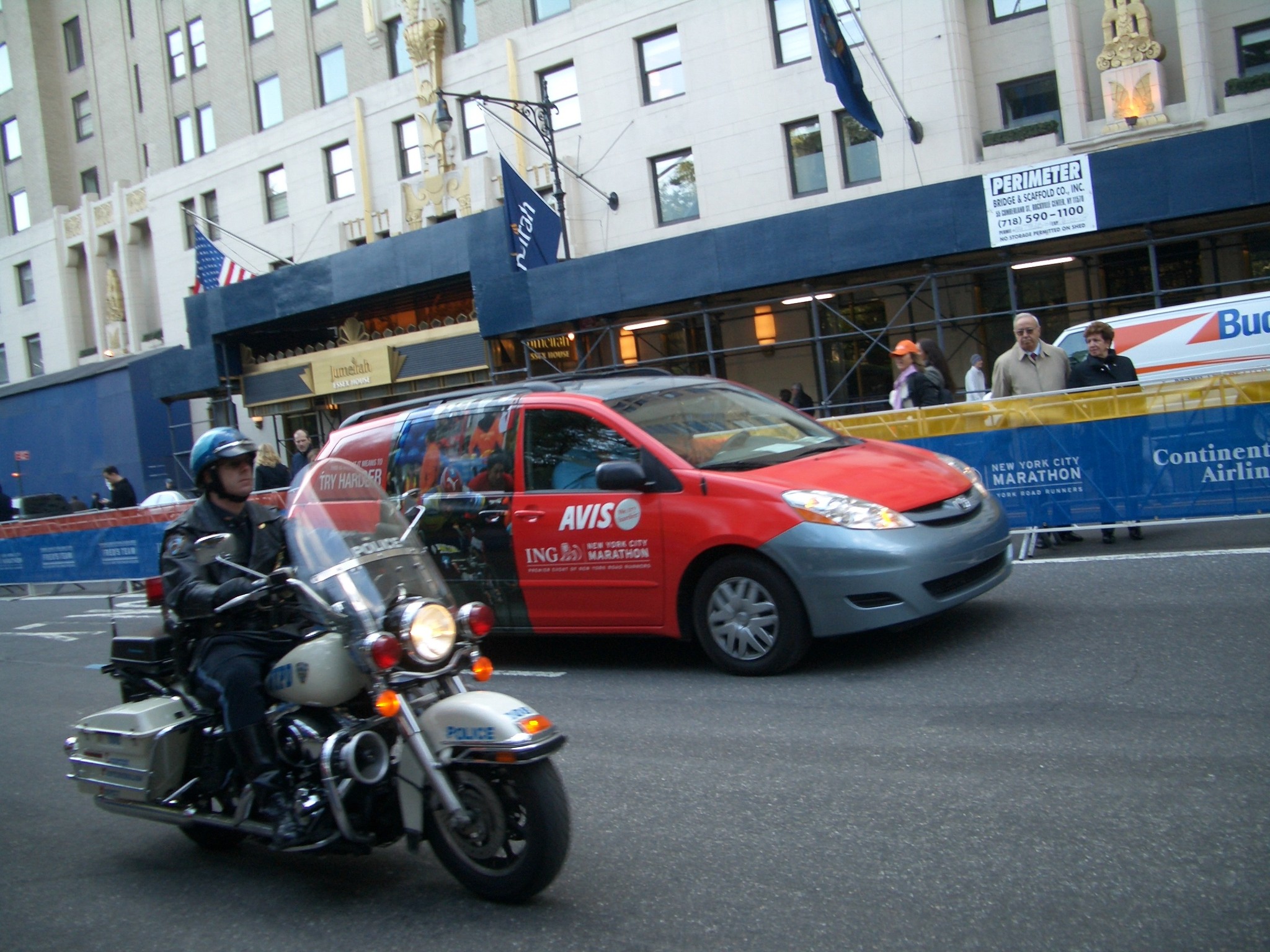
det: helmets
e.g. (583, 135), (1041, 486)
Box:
(440, 467), (462, 493)
(189, 426), (256, 479)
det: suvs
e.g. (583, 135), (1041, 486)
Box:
(281, 368), (1017, 677)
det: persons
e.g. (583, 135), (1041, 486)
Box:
(291, 430), (311, 478)
(91, 493), (104, 510)
(255, 443), (294, 491)
(551, 414), (628, 491)
(68, 496), (89, 512)
(306, 448), (320, 464)
(889, 339), (957, 409)
(778, 388), (792, 405)
(965, 354), (986, 401)
(989, 313), (1084, 549)
(1066, 322), (1143, 544)
(0, 485), (12, 522)
(161, 426), (371, 848)
(386, 401), (520, 606)
(97, 466), (137, 509)
(790, 382), (815, 416)
(163, 478), (173, 491)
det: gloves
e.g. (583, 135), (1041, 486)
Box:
(212, 577), (254, 611)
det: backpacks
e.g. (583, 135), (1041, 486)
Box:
(917, 375), (953, 407)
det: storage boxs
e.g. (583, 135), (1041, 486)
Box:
(109, 628), (177, 676)
(76, 697), (204, 802)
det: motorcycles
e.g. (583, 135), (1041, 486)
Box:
(60, 452), (573, 904)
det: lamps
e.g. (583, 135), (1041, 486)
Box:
(252, 416), (263, 429)
(435, 79), (572, 262)
(754, 306), (776, 358)
(619, 328), (641, 375)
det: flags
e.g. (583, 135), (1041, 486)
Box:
(498, 147), (562, 271)
(189, 223), (260, 294)
(804, 0), (884, 138)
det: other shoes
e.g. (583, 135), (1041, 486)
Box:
(1102, 535), (1116, 544)
(1034, 532), (1051, 549)
(1055, 531), (1084, 543)
(1128, 531), (1144, 540)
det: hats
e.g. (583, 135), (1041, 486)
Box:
(890, 339), (919, 355)
(969, 353), (981, 365)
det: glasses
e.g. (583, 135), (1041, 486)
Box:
(219, 454), (254, 470)
(1013, 328), (1034, 336)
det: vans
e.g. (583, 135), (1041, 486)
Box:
(1050, 288), (1270, 411)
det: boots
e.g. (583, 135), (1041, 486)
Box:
(225, 719), (303, 826)
